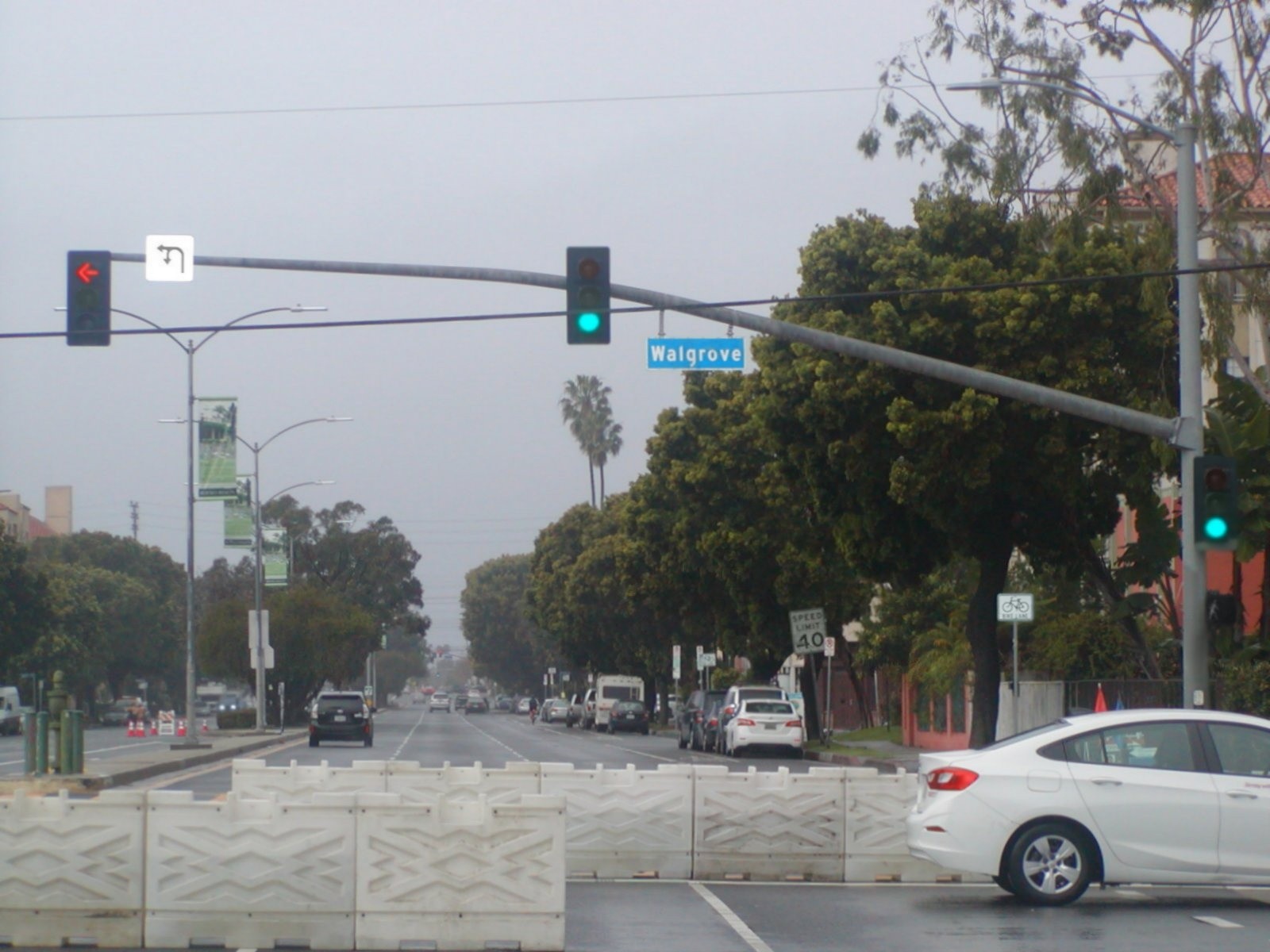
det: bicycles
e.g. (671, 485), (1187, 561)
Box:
(531, 710), (538, 724)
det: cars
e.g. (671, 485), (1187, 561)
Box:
(565, 692), (588, 729)
(606, 698), (652, 735)
(544, 698), (570, 724)
(922, 703), (1270, 909)
(720, 697), (806, 760)
(103, 695), (152, 727)
(653, 693), (680, 720)
(413, 685), (557, 722)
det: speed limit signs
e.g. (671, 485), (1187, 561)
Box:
(789, 607), (827, 655)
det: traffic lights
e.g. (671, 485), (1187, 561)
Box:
(65, 250), (111, 346)
(1192, 455), (1242, 546)
(565, 245), (611, 345)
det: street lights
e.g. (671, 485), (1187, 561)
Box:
(54, 305), (333, 743)
(288, 517), (356, 587)
(947, 76), (1215, 710)
(160, 416), (351, 731)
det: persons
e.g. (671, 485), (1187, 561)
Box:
(529, 695), (537, 718)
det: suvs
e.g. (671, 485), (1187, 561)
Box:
(308, 690), (377, 747)
(675, 684), (792, 755)
(577, 688), (598, 730)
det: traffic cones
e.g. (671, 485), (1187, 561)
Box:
(201, 718), (210, 732)
(177, 716), (187, 737)
(126, 716), (158, 737)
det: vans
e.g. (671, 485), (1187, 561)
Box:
(0, 686), (27, 736)
(195, 681), (272, 729)
(588, 674), (646, 732)
